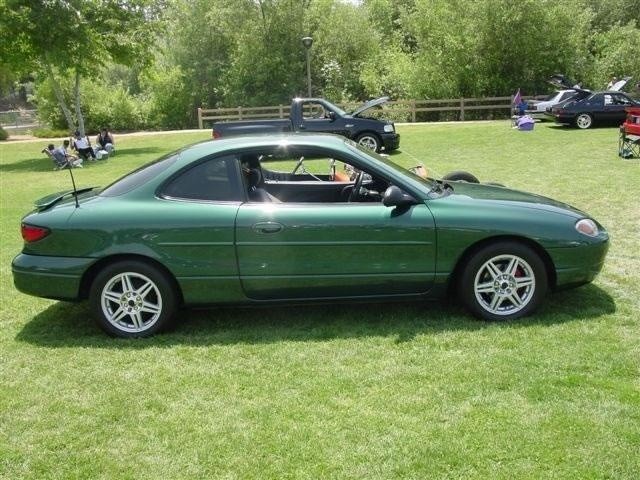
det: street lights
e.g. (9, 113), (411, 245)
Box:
(301, 37), (314, 118)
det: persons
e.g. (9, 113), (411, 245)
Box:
(47, 125), (116, 168)
(516, 99), (528, 116)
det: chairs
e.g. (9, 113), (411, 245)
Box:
(244, 169), (281, 201)
(41, 149), (83, 170)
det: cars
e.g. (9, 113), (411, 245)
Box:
(11, 129), (611, 340)
(523, 73), (640, 129)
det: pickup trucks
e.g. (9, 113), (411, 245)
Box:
(211, 94), (401, 155)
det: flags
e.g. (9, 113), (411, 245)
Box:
(514, 92), (522, 106)
